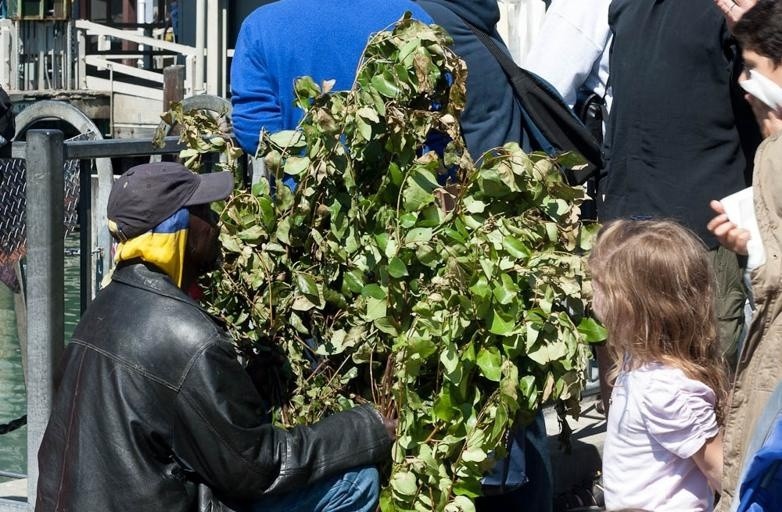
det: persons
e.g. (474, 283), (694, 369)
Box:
(35, 161), (399, 512)
(229, 0), (781, 511)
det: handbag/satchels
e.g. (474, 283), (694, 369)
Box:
(482, 407), (553, 512)
(575, 91), (603, 146)
(508, 67), (601, 186)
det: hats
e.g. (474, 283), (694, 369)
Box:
(108, 162), (234, 244)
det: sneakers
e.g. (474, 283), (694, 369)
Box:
(595, 399), (607, 414)
(557, 470), (605, 512)
